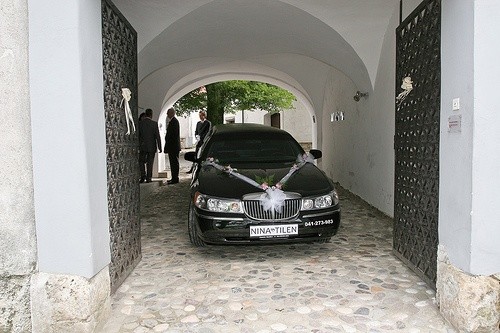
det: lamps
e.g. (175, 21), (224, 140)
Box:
(354, 91), (367, 101)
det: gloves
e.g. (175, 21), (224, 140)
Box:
(196, 135), (200, 141)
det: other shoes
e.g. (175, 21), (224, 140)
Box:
(146, 179), (152, 183)
(167, 180), (179, 184)
(139, 177), (145, 183)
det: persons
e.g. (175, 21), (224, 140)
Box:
(138, 109), (162, 183)
(185, 111), (212, 174)
(165, 107), (181, 184)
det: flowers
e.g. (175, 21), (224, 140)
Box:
(292, 161), (299, 170)
(223, 165), (234, 173)
(401, 77), (412, 92)
(254, 169), (283, 191)
(122, 88), (132, 102)
(206, 158), (219, 165)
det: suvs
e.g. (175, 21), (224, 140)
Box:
(183, 123), (343, 248)
